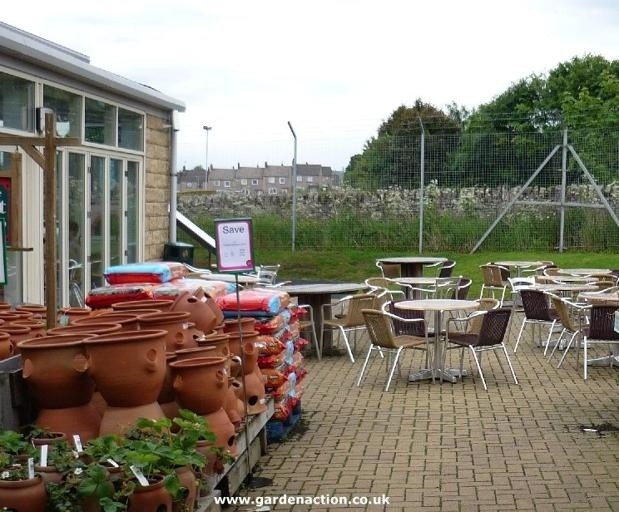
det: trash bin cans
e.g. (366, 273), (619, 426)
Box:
(163, 242), (194, 266)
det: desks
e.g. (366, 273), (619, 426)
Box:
(375, 257), (450, 300)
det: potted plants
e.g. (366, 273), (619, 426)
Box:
(1, 409), (237, 512)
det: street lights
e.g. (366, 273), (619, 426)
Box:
(201, 126), (213, 189)
